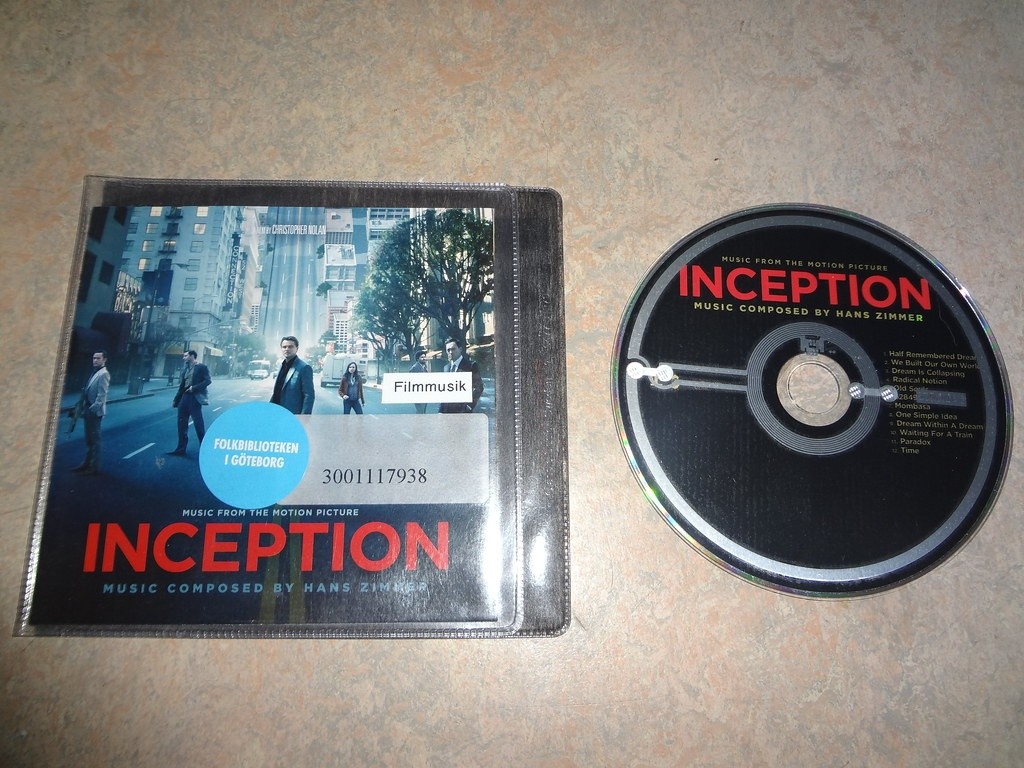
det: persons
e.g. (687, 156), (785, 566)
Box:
(70, 349), (110, 475)
(338, 362), (364, 414)
(411, 350), (430, 414)
(438, 338), (484, 413)
(165, 349), (212, 456)
(266, 336), (315, 415)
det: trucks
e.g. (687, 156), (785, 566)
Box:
(322, 352), (356, 386)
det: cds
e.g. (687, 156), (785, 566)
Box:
(610, 204), (1015, 600)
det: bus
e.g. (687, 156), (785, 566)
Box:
(247, 361), (270, 381)
